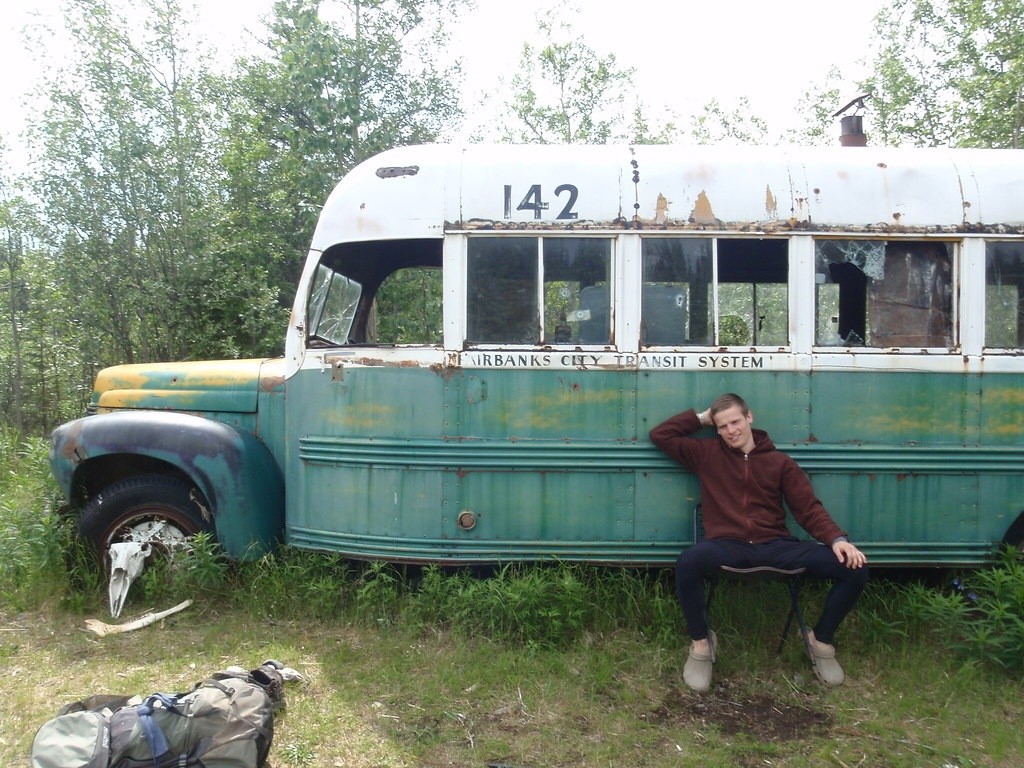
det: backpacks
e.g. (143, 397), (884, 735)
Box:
(30, 671), (272, 768)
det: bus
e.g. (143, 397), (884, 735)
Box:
(47, 144), (1024, 679)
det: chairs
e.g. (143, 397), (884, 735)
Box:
(694, 502), (816, 669)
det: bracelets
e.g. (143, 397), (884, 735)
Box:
(697, 413), (704, 425)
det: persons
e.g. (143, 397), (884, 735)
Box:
(649, 392), (869, 691)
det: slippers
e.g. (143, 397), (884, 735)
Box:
(683, 631), (717, 691)
(798, 626), (844, 687)
